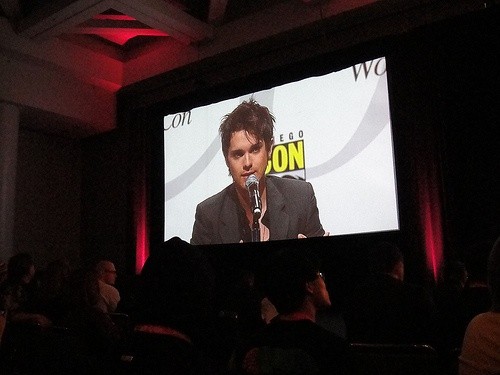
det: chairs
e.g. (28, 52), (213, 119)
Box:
(10, 310), (437, 375)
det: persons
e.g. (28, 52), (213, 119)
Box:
(0, 237), (500, 375)
(190, 100), (329, 245)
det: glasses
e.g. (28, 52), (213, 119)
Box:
(312, 272), (326, 280)
(104, 270), (117, 274)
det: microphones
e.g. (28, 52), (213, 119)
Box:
(245, 174), (262, 219)
